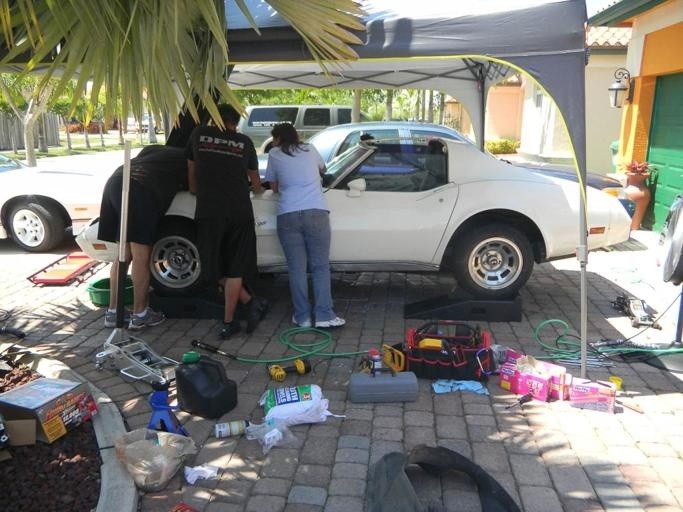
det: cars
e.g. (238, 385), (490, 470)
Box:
(0, 149), (108, 253)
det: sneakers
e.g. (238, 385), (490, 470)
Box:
(128, 308), (167, 330)
(104, 306), (132, 327)
(292, 313), (313, 327)
(222, 319), (241, 340)
(315, 317), (346, 328)
(242, 295), (269, 332)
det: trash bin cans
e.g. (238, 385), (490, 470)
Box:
(609, 139), (620, 172)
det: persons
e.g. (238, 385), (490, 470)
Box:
(184, 104), (271, 340)
(97, 134), (191, 332)
(266, 123), (346, 329)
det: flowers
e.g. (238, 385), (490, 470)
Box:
(622, 159), (657, 174)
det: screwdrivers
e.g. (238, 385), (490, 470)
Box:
(505, 393), (532, 409)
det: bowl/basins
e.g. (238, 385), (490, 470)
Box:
(86, 277), (133, 305)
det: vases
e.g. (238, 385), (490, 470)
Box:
(3, 419), (37, 446)
(625, 171), (652, 230)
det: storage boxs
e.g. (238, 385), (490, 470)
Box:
(0, 377), (98, 444)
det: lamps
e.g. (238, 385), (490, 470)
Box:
(608, 67), (635, 109)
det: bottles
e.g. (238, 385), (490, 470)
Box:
(369, 353), (382, 376)
(214, 419), (249, 438)
